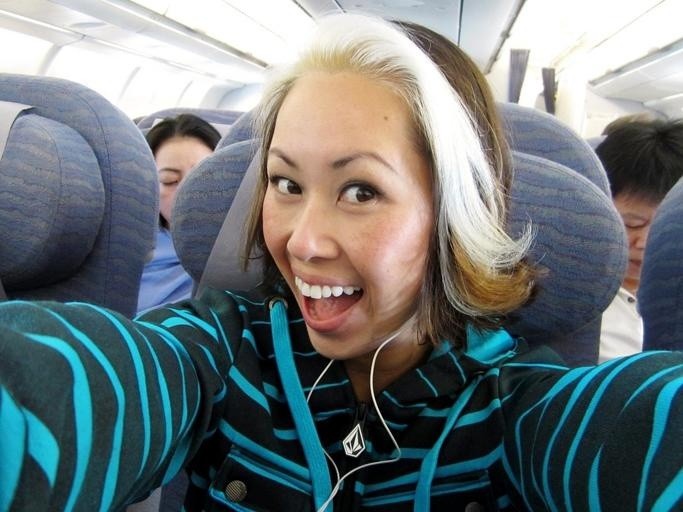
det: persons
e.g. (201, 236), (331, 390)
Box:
(593, 119), (683, 365)
(0, 12), (683, 512)
(136, 116), (221, 312)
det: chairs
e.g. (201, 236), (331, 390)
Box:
(0, 74), (159, 320)
(170, 95), (630, 367)
(638, 176), (683, 353)
